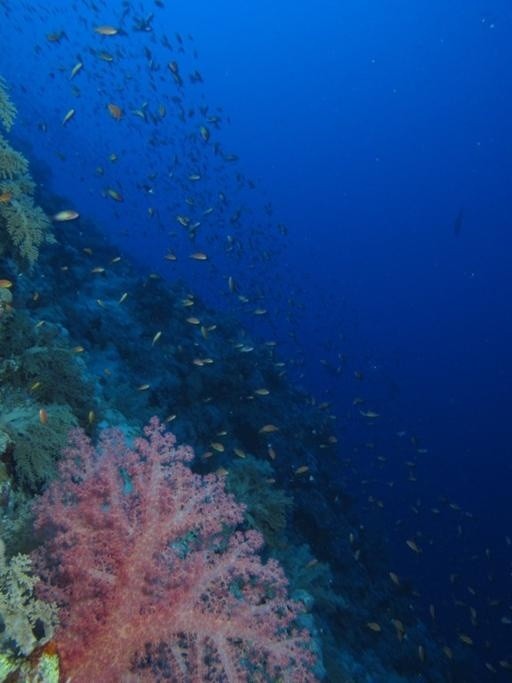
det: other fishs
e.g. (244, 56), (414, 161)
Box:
(2, 3), (307, 345)
(1, 349), (511, 676)
